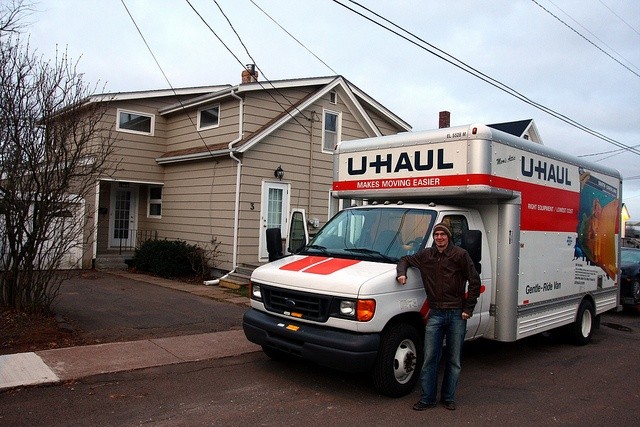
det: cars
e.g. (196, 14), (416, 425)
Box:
(618, 244), (640, 309)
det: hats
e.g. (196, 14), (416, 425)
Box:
(433, 224), (452, 238)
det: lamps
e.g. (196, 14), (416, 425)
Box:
(275, 165), (284, 182)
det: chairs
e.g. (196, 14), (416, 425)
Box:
(374, 230), (400, 255)
(411, 237), (426, 247)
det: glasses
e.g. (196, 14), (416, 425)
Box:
(434, 233), (447, 236)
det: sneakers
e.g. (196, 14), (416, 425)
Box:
(413, 402), (436, 411)
(440, 400), (456, 410)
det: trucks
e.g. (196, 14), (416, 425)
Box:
(240, 121), (624, 398)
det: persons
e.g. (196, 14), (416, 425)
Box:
(396, 223), (481, 411)
